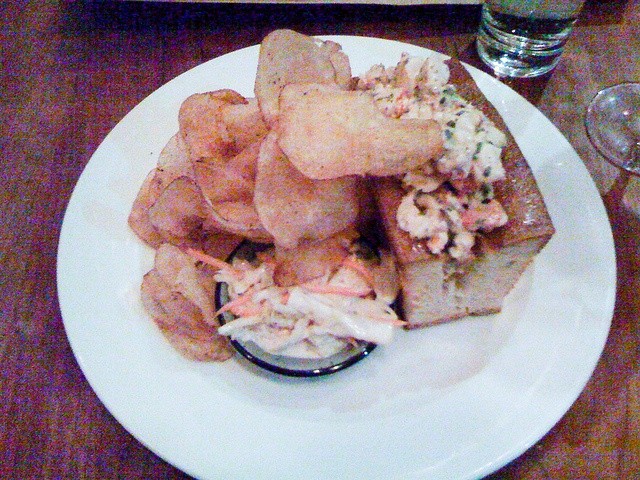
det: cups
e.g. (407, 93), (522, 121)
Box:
(476, 0), (586, 78)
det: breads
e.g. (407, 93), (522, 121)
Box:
(349, 54), (555, 330)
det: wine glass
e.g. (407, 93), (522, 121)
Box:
(584, 81), (640, 177)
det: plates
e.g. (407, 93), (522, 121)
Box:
(213, 239), (398, 377)
(55, 33), (620, 480)
